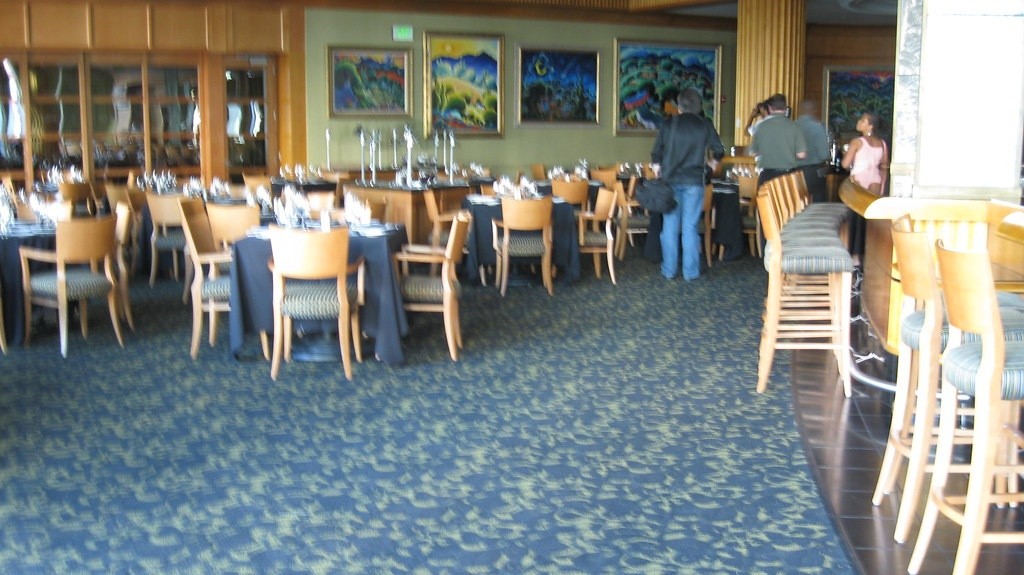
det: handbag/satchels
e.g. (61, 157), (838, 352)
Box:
(635, 178), (677, 214)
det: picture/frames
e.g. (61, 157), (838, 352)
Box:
(822, 65), (895, 168)
(513, 41), (604, 130)
(326, 45), (414, 121)
(421, 29), (506, 140)
(612, 37), (724, 138)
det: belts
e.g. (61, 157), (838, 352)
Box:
(764, 168), (796, 172)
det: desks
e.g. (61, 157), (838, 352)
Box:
(711, 181), (740, 203)
(230, 220), (410, 369)
(712, 188), (744, 263)
(0, 220), (54, 348)
(268, 176), (338, 210)
(530, 179), (606, 213)
(463, 193), (582, 289)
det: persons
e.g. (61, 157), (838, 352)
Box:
(746, 92), (829, 263)
(841, 112), (889, 266)
(651, 90), (724, 280)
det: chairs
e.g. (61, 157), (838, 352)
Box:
(737, 170), (853, 400)
(57, 182), (104, 217)
(267, 224), (366, 381)
(52, 201), (133, 340)
(491, 162), (713, 298)
(104, 180), (162, 275)
(423, 187), (486, 287)
(205, 202), (261, 273)
(17, 209), (126, 358)
(242, 172), (273, 199)
(178, 197), (272, 361)
(394, 212), (470, 362)
(906, 238), (1024, 575)
(144, 188), (203, 290)
(870, 214), (1024, 542)
